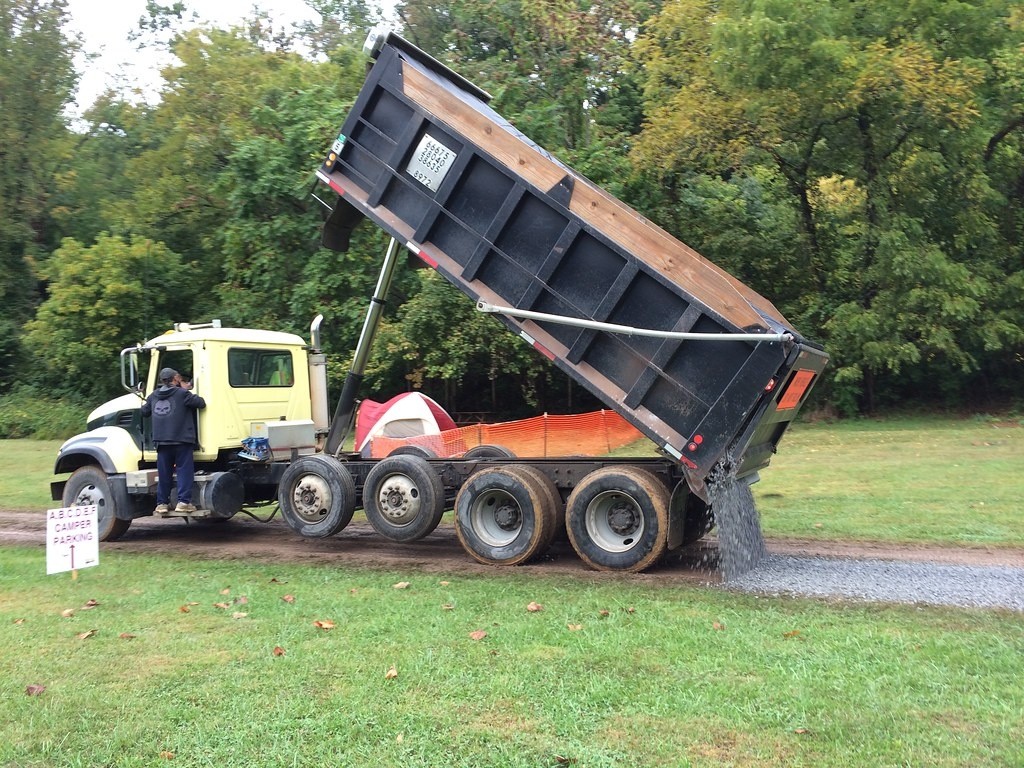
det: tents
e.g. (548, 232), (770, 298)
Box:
(354, 391), (469, 459)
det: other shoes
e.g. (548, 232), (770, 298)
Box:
(155, 504), (169, 512)
(175, 503), (197, 512)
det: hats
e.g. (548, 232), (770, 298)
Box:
(160, 368), (177, 380)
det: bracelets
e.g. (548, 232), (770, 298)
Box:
(179, 379), (183, 384)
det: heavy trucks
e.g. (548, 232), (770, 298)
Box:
(50, 19), (832, 574)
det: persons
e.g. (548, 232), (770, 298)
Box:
(177, 373), (194, 390)
(141, 367), (206, 513)
